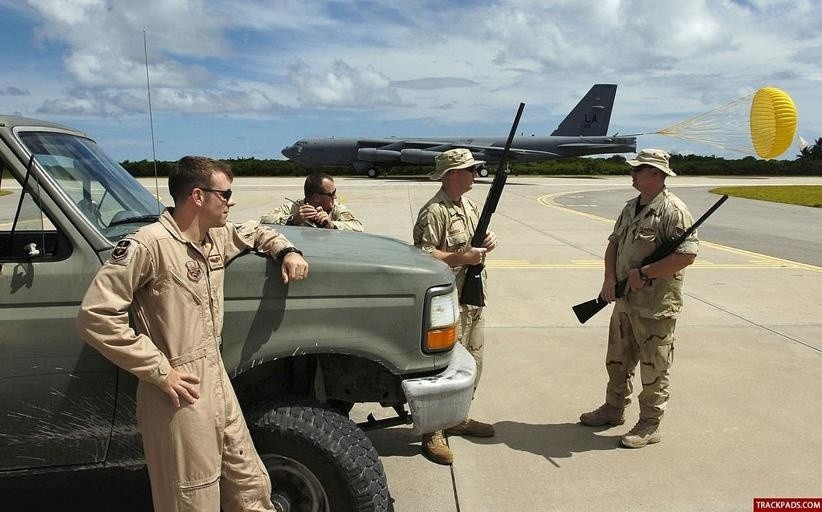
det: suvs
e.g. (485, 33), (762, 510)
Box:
(0, 113), (478, 509)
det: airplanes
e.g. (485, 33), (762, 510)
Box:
(281, 83), (638, 180)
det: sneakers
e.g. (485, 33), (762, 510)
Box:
(580, 403), (626, 426)
(620, 427), (661, 449)
(445, 419), (495, 437)
(421, 432), (453, 464)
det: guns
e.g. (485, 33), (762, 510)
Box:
(571, 193), (729, 324)
(459, 103), (527, 307)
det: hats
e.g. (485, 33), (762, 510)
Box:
(625, 149), (677, 177)
(429, 148), (486, 180)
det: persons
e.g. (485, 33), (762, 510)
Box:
(75, 155), (310, 512)
(260, 172), (364, 233)
(412, 148), (497, 466)
(578, 148), (699, 450)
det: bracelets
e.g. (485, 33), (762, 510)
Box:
(277, 246), (303, 263)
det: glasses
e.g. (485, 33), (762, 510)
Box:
(466, 166), (476, 173)
(206, 188), (232, 200)
(632, 166), (640, 172)
(322, 188), (336, 196)
